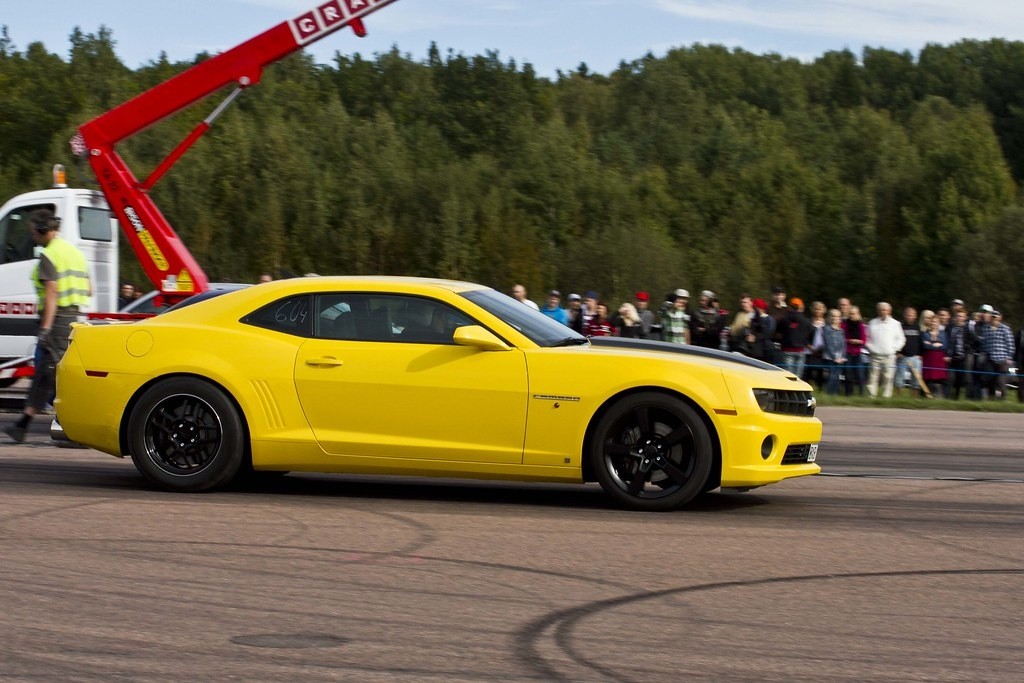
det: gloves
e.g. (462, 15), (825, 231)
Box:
(39, 327), (52, 350)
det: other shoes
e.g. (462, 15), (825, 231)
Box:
(0, 423), (26, 442)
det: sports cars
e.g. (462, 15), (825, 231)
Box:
(49, 274), (826, 511)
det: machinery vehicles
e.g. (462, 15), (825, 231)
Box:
(1, 1), (401, 405)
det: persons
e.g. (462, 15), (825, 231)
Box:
(258, 271), (274, 283)
(119, 284), (153, 313)
(512, 284), (1024, 402)
(397, 301), (466, 341)
(0, 210), (92, 443)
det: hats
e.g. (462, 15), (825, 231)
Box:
(568, 294), (581, 300)
(752, 299), (768, 309)
(980, 304), (993, 312)
(953, 299), (964, 306)
(791, 298), (803, 306)
(675, 289), (691, 299)
(550, 290), (561, 297)
(636, 292), (648, 300)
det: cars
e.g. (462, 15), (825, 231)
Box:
(117, 280), (401, 336)
